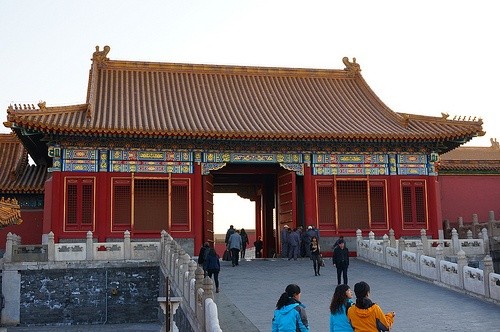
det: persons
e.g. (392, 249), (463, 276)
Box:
(329, 284), (355, 332)
(333, 240), (349, 284)
(347, 281), (395, 332)
(282, 225), (320, 260)
(198, 242), (212, 277)
(205, 247), (220, 292)
(240, 229), (249, 258)
(225, 225), (236, 246)
(254, 236), (263, 258)
(271, 230), (276, 258)
(310, 235), (322, 276)
(332, 235), (346, 248)
(228, 230), (242, 267)
(272, 284), (310, 332)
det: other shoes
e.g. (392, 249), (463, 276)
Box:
(236, 263), (239, 266)
(216, 290), (219, 293)
(315, 273), (317, 276)
(232, 265), (235, 267)
(318, 272), (320, 275)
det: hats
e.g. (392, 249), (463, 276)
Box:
(308, 226), (312, 229)
(284, 225), (289, 228)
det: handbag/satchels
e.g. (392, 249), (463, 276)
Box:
(320, 259), (326, 267)
(222, 250), (232, 261)
